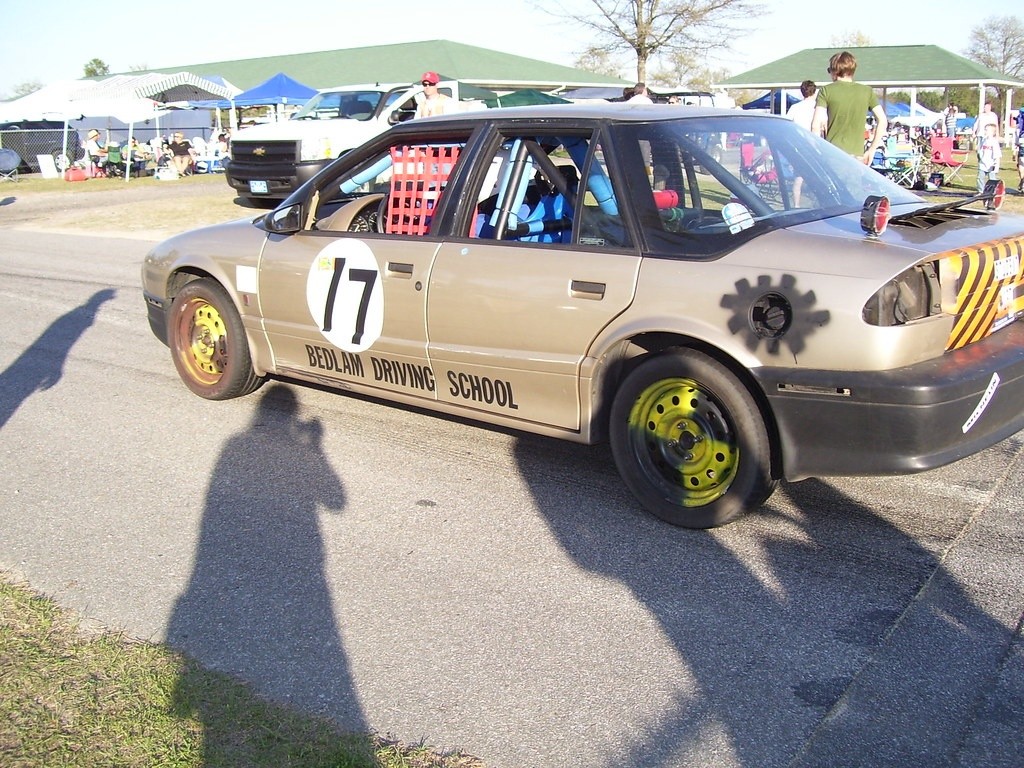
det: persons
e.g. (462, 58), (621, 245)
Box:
(942, 100), (958, 140)
(167, 131), (196, 178)
(85, 128), (117, 177)
(811, 51), (888, 203)
(412, 71), (460, 187)
(122, 137), (151, 177)
(214, 133), (229, 169)
(972, 101), (1002, 196)
(1012, 105), (1024, 197)
(623, 81), (712, 186)
(786, 80), (828, 209)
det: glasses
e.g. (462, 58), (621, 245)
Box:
(423, 82), (436, 86)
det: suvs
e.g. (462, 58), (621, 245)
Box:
(0, 118), (85, 174)
(226, 70), (737, 212)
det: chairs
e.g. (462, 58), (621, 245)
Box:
(740, 139), (811, 201)
(475, 150), (580, 243)
(168, 137), (222, 175)
(864, 137), (971, 188)
(0, 148), (22, 185)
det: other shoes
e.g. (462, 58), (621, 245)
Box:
(974, 192), (983, 198)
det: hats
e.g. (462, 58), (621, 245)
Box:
(88, 130), (98, 139)
(422, 71), (439, 83)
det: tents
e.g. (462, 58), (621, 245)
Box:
(133, 71), (236, 178)
(473, 88), (574, 110)
(84, 72), (174, 182)
(317, 86), (402, 112)
(0, 71), (99, 178)
(743, 88), (977, 142)
(217, 71), (325, 130)
(153, 77), (244, 162)
(413, 73), (501, 108)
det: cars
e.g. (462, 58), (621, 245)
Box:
(140, 98), (1024, 531)
